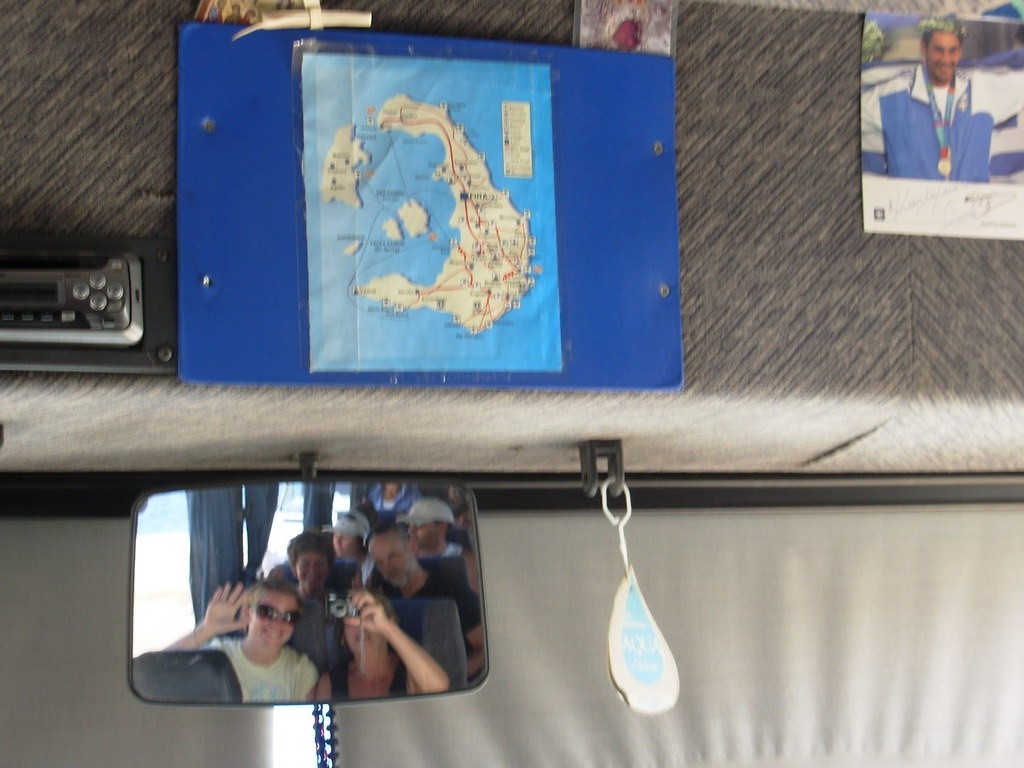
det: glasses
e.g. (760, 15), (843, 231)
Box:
(248, 603), (299, 624)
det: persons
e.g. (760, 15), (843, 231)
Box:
(862, 14), (994, 182)
(163, 473), (485, 703)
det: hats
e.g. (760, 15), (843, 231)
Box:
(395, 496), (454, 528)
(322, 517), (370, 539)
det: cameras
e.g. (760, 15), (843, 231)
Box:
(325, 593), (359, 622)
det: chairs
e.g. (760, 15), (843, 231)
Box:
(133, 650), (243, 705)
(416, 556), (480, 619)
(276, 557), (359, 599)
(293, 595), (340, 673)
(388, 599), (468, 692)
